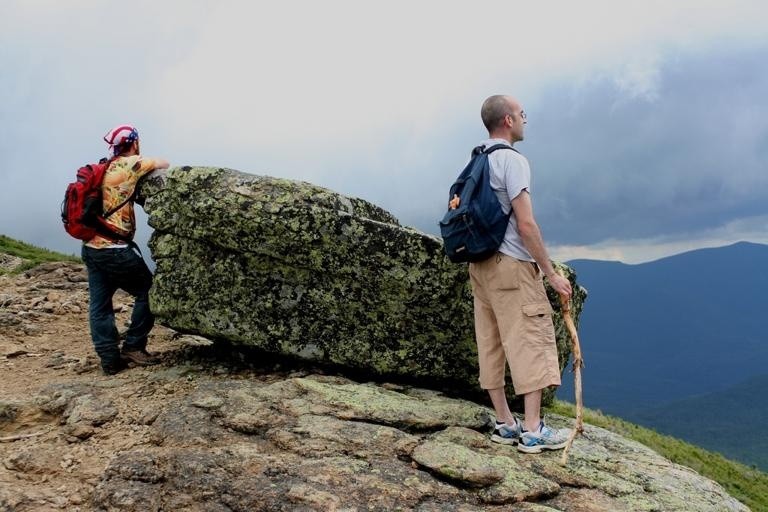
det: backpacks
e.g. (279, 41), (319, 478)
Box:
(438, 144), (521, 264)
(61, 156), (120, 244)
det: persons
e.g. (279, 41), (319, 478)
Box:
(80, 124), (171, 376)
(467, 93), (575, 454)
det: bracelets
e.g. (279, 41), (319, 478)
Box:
(547, 271), (557, 279)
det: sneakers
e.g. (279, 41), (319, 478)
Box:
(490, 415), (525, 446)
(120, 345), (159, 366)
(517, 422), (574, 454)
(102, 356), (123, 374)
